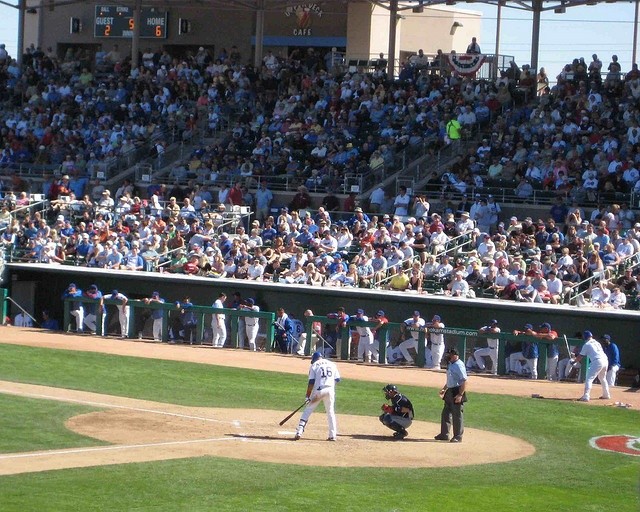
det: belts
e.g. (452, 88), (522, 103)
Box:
(248, 324), (255, 326)
(490, 346), (495, 349)
(414, 339), (418, 340)
(361, 334), (369, 337)
(432, 342), (440, 345)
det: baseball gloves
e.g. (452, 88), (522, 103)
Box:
(381, 403), (394, 414)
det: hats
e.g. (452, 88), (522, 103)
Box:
(408, 217), (416, 223)
(355, 207), (363, 212)
(523, 324), (532, 330)
(333, 254), (341, 258)
(512, 253), (523, 259)
(252, 220), (260, 225)
(102, 190), (110, 195)
(311, 352), (322, 363)
(383, 214), (390, 218)
(244, 298), (254, 304)
(83, 234), (89, 239)
(152, 291), (159, 296)
(91, 285), (96, 289)
(621, 235), (628, 239)
(57, 215), (64, 220)
(432, 315), (441, 320)
(206, 246), (215, 253)
(599, 334), (611, 340)
(492, 320), (497, 324)
(375, 310), (385, 316)
(112, 290), (118, 295)
(538, 224), (545, 229)
(582, 331), (592, 339)
(540, 323), (551, 329)
(253, 257), (259, 261)
(413, 311), (420, 316)
(582, 220), (589, 225)
(356, 309), (364, 314)
(461, 212), (469, 217)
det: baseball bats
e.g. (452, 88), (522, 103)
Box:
(279, 399), (310, 426)
(563, 334), (572, 364)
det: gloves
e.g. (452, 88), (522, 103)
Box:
(570, 360), (575, 365)
(305, 397), (310, 404)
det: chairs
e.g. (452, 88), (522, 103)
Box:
(335, 240), (361, 260)
(481, 286), (495, 298)
(422, 276), (435, 292)
(435, 279), (447, 292)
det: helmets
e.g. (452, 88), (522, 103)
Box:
(442, 348), (459, 365)
(383, 384), (397, 400)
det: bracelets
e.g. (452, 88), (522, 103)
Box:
(459, 393), (463, 397)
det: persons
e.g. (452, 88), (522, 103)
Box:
(399, 311), (427, 364)
(570, 331), (610, 402)
(211, 292), (227, 347)
(509, 329), (525, 376)
(601, 334), (620, 386)
(14, 309), (33, 326)
(466, 347), (482, 373)
(525, 323), (557, 382)
(438, 194), (573, 304)
(100, 290), (130, 338)
(367, 310), (388, 365)
(141, 290), (165, 342)
(40, 309), (60, 329)
(262, 186), (446, 292)
(175, 295), (197, 344)
(554, 334), (570, 381)
(83, 284), (106, 336)
(226, 291), (245, 350)
(275, 308), (294, 354)
(1, 172), (264, 282)
(414, 315), (445, 370)
(296, 309), (320, 356)
(61, 283), (84, 332)
(434, 347), (467, 443)
(385, 337), (403, 365)
(379, 383), (414, 439)
(557, 332), (584, 379)
(474, 319), (501, 375)
(327, 307), (352, 359)
(239, 298), (260, 351)
(288, 313), (305, 354)
(295, 351), (341, 441)
(341, 308), (374, 363)
(513, 324), (539, 380)
(563, 204), (640, 309)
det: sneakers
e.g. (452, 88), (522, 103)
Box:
(393, 427), (405, 435)
(577, 397), (589, 401)
(327, 436), (336, 441)
(295, 433), (301, 439)
(450, 436), (462, 442)
(435, 434), (448, 440)
(395, 431), (408, 439)
(599, 396), (605, 399)
